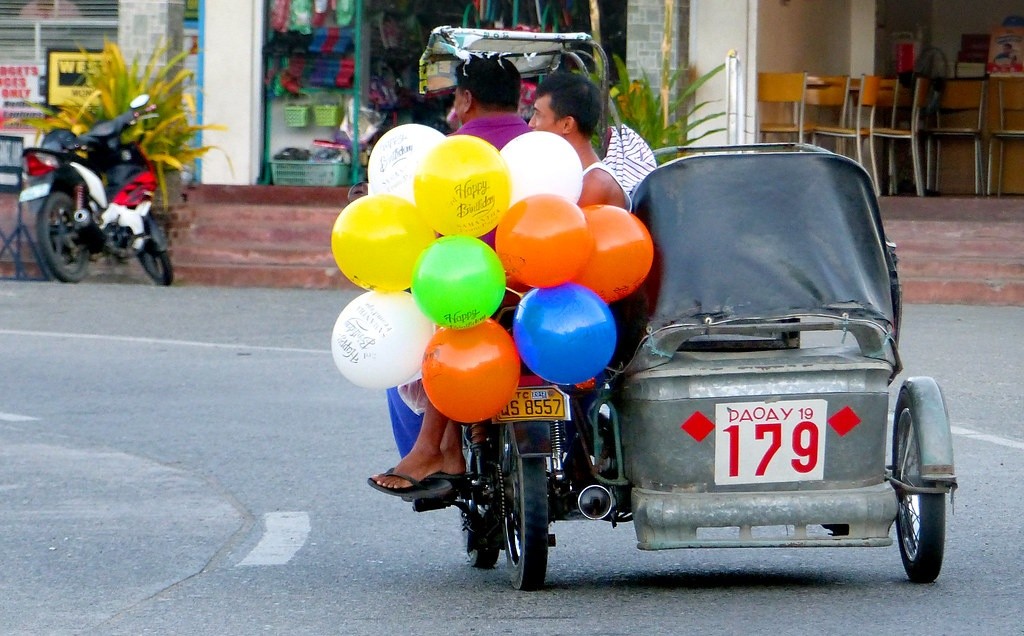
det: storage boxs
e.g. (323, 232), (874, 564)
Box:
(267, 159), (351, 189)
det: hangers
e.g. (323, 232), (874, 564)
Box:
(266, 57), (355, 94)
(262, 28), (353, 58)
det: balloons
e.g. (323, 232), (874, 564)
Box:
(330, 121), (654, 422)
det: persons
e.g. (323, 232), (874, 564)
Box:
(387, 52), (535, 464)
(367, 69), (633, 498)
(994, 43), (1017, 65)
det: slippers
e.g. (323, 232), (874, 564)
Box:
(367, 467), (472, 499)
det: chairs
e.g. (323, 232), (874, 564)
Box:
(756, 70), (1024, 198)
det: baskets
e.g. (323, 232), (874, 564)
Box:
(269, 159), (352, 186)
(284, 91), (345, 127)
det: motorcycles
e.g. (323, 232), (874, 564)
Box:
(18, 94), (175, 285)
(413, 24), (960, 592)
